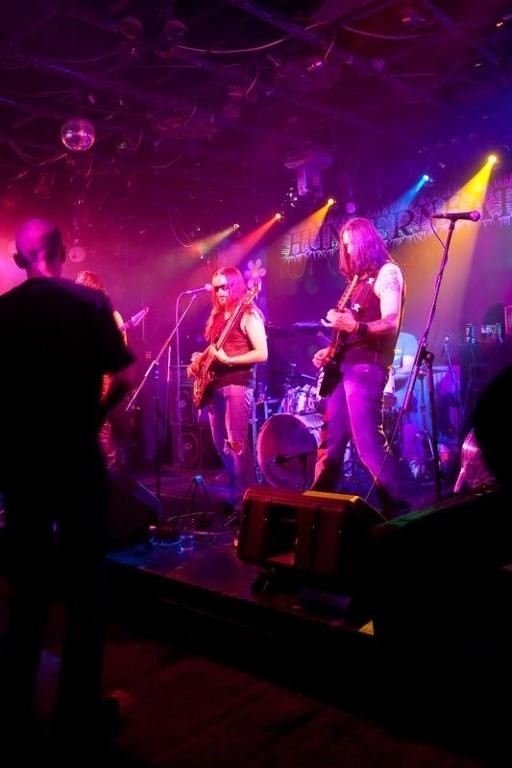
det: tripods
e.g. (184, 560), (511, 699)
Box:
(175, 473), (223, 531)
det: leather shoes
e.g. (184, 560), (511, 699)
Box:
(213, 471), (230, 484)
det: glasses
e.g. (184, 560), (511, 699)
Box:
(214, 284), (230, 292)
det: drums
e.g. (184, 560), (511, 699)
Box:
(382, 371), (396, 408)
(255, 408), (327, 490)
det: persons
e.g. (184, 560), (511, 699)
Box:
(304, 217), (430, 518)
(1, 216), (133, 748)
(76, 270), (130, 465)
(382, 331), (424, 477)
(434, 301), (504, 488)
(185, 265), (270, 526)
(474, 358), (510, 484)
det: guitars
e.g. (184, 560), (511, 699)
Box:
(317, 274), (357, 395)
(193, 280), (259, 409)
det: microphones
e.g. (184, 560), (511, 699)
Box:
(441, 337), (449, 357)
(183, 282), (213, 296)
(432, 210), (481, 222)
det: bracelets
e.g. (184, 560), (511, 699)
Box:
(351, 321), (368, 337)
(227, 356), (236, 367)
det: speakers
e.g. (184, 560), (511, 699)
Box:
(108, 461), (162, 545)
(237, 482), (388, 573)
